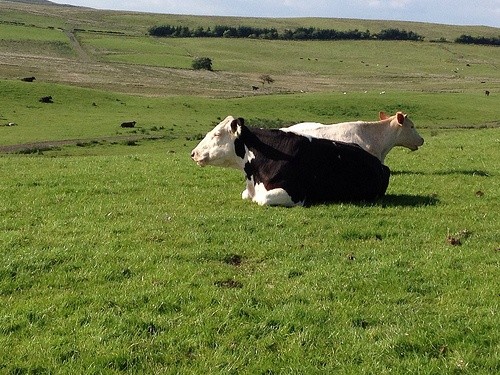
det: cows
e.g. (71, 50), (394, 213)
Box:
(190, 114), (391, 208)
(279, 110), (425, 165)
